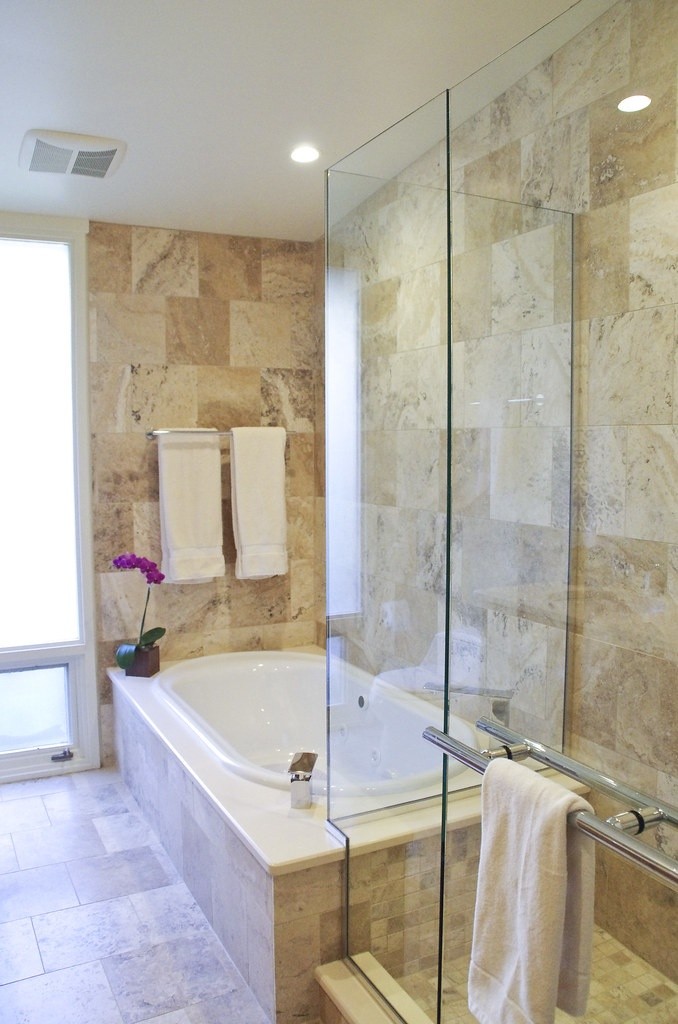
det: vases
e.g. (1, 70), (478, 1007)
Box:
(126, 645), (161, 678)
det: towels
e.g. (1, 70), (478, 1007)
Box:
(156, 427), (226, 584)
(229, 426), (289, 581)
(467, 755), (597, 1022)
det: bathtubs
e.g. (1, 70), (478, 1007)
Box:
(104, 642), (595, 1023)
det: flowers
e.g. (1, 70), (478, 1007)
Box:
(112, 553), (167, 670)
